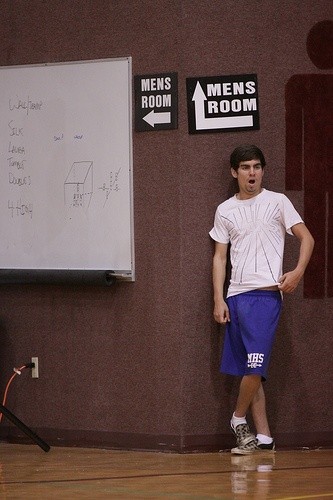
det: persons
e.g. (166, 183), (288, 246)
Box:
(230, 452), (276, 500)
(208, 145), (314, 455)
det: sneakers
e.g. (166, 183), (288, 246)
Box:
(231, 439), (276, 455)
(228, 420), (256, 450)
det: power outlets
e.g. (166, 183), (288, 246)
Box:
(31, 357), (39, 379)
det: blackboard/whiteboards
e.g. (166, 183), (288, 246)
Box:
(0, 56), (135, 286)
